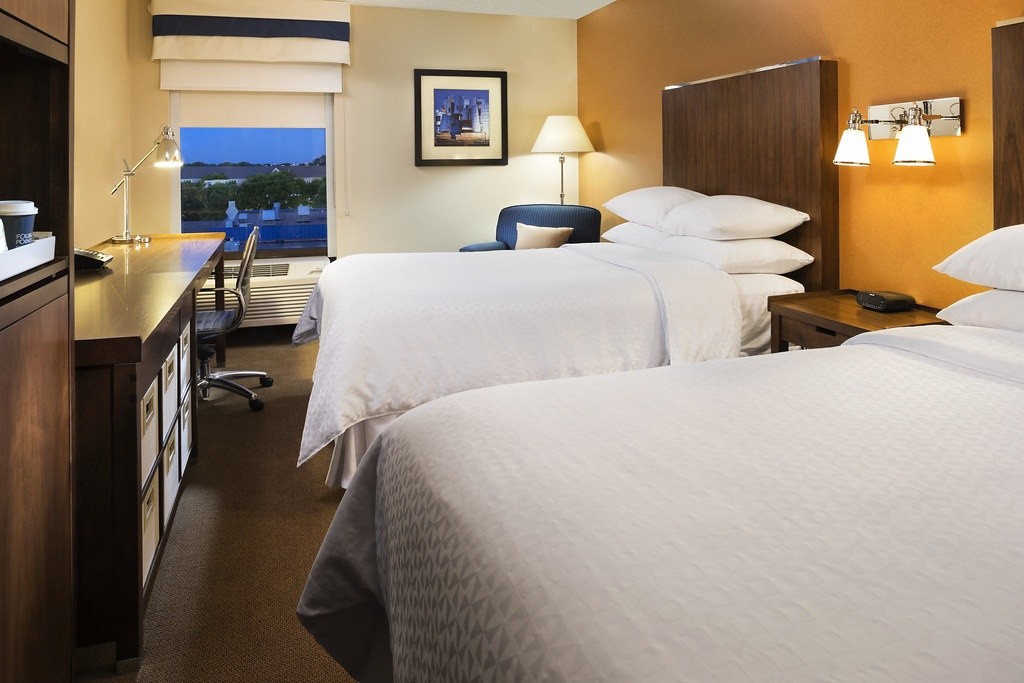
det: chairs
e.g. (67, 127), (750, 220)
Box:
(196, 226), (274, 411)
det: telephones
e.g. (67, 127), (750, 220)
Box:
(74, 247), (114, 269)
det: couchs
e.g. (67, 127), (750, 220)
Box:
(458, 204), (601, 252)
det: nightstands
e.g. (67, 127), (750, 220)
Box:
(767, 288), (952, 355)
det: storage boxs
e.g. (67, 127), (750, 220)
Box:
(0, 232), (54, 280)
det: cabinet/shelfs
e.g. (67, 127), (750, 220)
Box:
(72, 233), (226, 658)
(0, 0), (75, 683)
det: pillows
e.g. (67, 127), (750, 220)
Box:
(601, 221), (814, 274)
(936, 289), (1024, 332)
(515, 222), (574, 250)
(931, 223), (1024, 292)
(603, 185), (810, 240)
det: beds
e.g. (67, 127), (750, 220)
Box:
(293, 57), (839, 489)
(300, 18), (1024, 683)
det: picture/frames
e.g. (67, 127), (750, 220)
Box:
(414, 69), (509, 166)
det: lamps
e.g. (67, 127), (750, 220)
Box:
(111, 123), (183, 243)
(832, 96), (961, 167)
(531, 115), (595, 205)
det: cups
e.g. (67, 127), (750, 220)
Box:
(0, 200), (38, 251)
